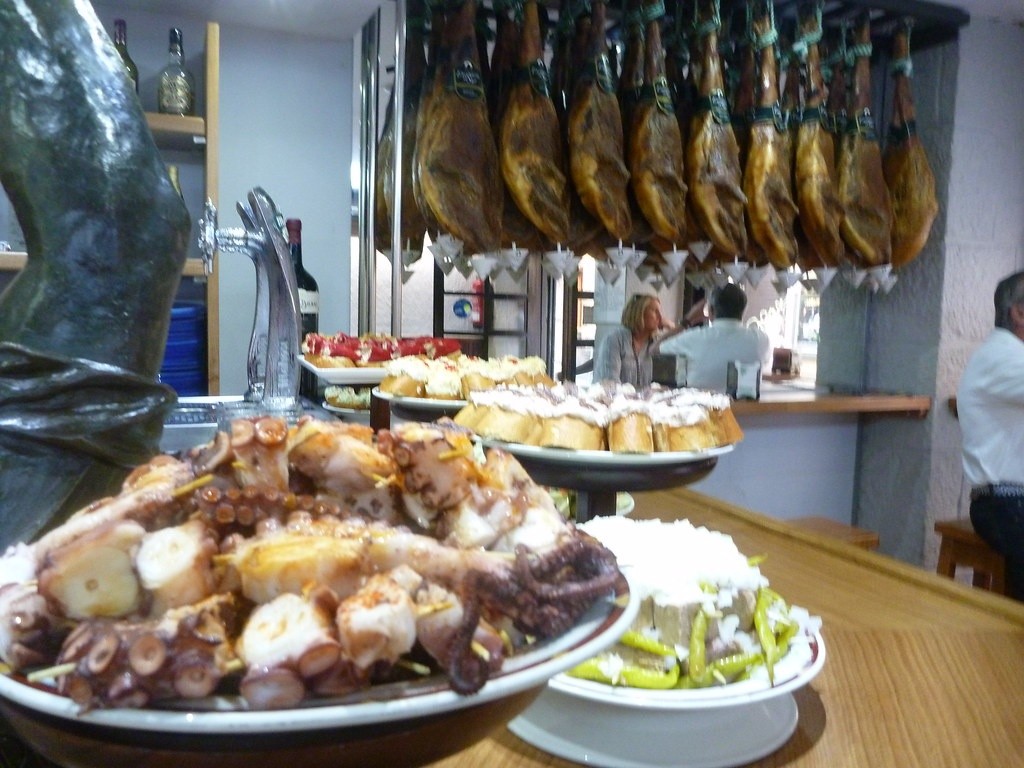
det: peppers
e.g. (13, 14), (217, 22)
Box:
(567, 554), (799, 692)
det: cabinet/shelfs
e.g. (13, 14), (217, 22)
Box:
(0, 21), (220, 396)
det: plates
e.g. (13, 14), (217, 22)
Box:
(321, 402), (370, 419)
(475, 434), (735, 464)
(298, 355), (379, 385)
(373, 386), (467, 410)
(0, 562), (640, 726)
(507, 692), (798, 768)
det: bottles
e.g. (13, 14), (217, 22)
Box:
(114, 19), (139, 92)
(286, 219), (319, 339)
(158, 28), (194, 115)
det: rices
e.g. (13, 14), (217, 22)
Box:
(582, 515), (824, 686)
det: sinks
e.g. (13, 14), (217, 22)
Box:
(158, 423), (223, 455)
(163, 400), (219, 425)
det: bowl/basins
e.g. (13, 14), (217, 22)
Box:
(547, 604), (828, 709)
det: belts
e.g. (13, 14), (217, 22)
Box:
(970, 484), (1024, 498)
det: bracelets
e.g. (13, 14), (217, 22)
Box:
(680, 317), (693, 328)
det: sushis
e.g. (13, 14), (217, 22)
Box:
(452, 380), (745, 454)
(300, 332), (557, 401)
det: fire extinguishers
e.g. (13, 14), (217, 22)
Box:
(470, 279), (483, 330)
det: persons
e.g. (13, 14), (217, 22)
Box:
(956, 270), (1024, 605)
(592, 292), (676, 388)
(647, 281), (772, 394)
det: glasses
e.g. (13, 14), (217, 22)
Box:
(632, 294), (640, 303)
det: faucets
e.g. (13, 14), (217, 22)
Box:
(197, 185), (303, 409)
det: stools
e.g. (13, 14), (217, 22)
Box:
(934, 517), (1005, 596)
(780, 516), (880, 551)
